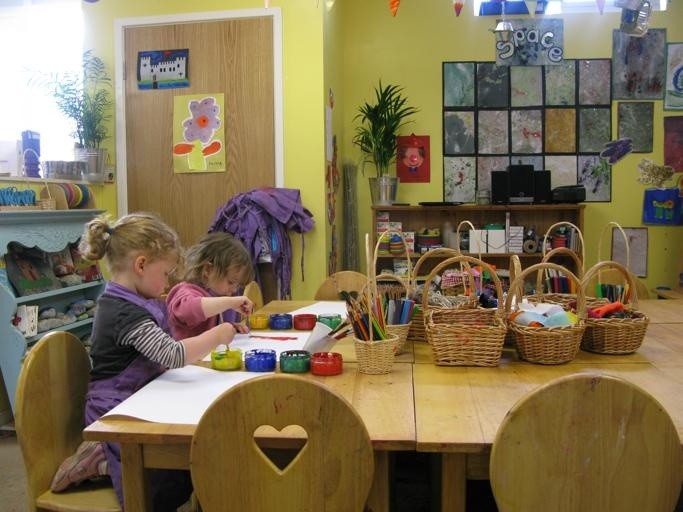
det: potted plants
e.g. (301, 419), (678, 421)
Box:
(352, 78), (422, 205)
(30, 46), (115, 179)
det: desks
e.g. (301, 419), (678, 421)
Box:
(200, 299), (414, 363)
(413, 340), (648, 363)
(624, 299), (683, 323)
(414, 363), (683, 512)
(80, 363), (414, 511)
(637, 324), (683, 385)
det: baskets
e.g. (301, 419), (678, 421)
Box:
(422, 256), (506, 368)
(359, 274), (412, 355)
(579, 260), (649, 355)
(500, 254), (524, 348)
(505, 262), (586, 365)
(529, 247), (594, 307)
(406, 246), (476, 343)
(352, 333), (399, 374)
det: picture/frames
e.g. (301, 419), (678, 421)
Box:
(544, 108), (578, 154)
(612, 29), (665, 100)
(618, 102), (653, 152)
(477, 158), (511, 203)
(478, 108), (510, 155)
(476, 61), (510, 109)
(442, 60), (475, 108)
(577, 58), (611, 107)
(579, 155), (611, 203)
(544, 59), (577, 106)
(510, 64), (544, 108)
(577, 108), (612, 154)
(664, 42), (683, 110)
(511, 156), (544, 171)
(443, 157), (476, 203)
(511, 109), (543, 155)
(443, 110), (476, 156)
(611, 227), (648, 278)
(545, 154), (578, 191)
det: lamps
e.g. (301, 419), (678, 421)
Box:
(620, 1), (650, 37)
(495, 2), (514, 32)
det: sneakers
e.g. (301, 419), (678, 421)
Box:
(50, 440), (105, 493)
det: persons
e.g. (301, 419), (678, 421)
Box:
(165, 235), (253, 346)
(51, 214), (237, 493)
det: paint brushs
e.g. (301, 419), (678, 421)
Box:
(381, 286), (420, 326)
(247, 316), (251, 337)
(220, 312), (230, 351)
(595, 281), (630, 304)
(329, 319), (355, 340)
(339, 291), (387, 342)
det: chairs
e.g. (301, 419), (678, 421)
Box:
(13, 332), (123, 512)
(240, 280), (264, 321)
(490, 372), (683, 511)
(190, 374), (376, 511)
(314, 271), (374, 301)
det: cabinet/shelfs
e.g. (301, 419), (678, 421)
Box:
(1, 177), (114, 432)
(370, 204), (587, 293)
